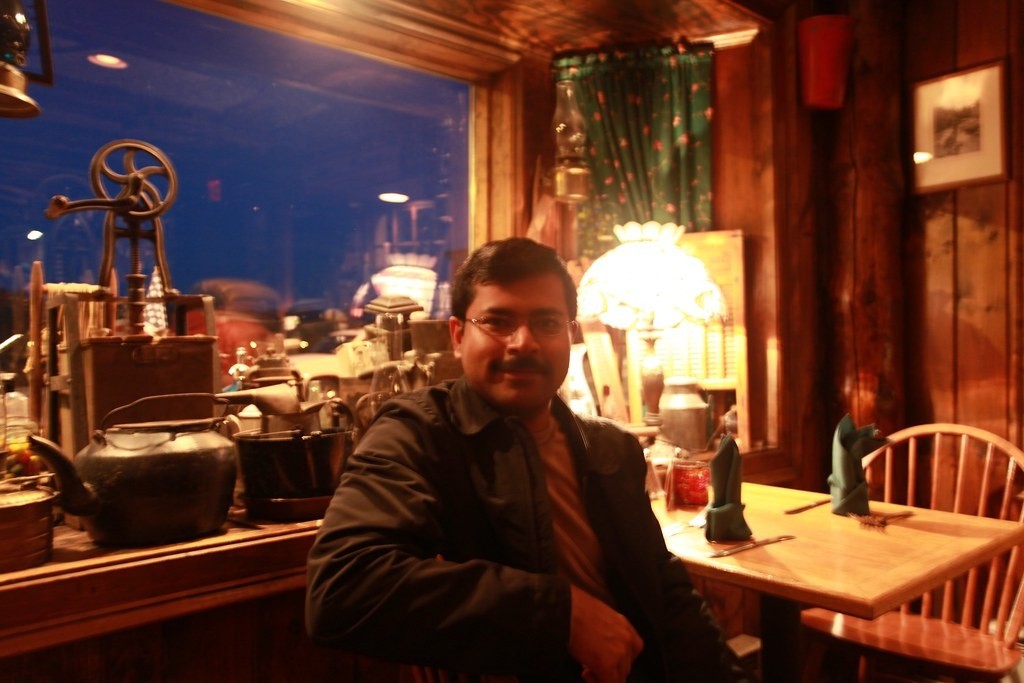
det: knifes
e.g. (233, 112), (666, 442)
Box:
(784, 498), (832, 515)
(709, 536), (795, 557)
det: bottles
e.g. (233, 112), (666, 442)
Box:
(657, 377), (711, 456)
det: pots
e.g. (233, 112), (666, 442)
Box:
(0, 472), (62, 572)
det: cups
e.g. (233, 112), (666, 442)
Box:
(665, 460), (711, 511)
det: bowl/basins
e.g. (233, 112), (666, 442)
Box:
(236, 491), (338, 522)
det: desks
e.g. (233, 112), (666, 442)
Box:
(622, 481), (1024, 683)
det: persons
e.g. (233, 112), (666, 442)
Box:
(306, 237), (725, 683)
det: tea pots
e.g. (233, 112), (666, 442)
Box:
(27, 393), (239, 549)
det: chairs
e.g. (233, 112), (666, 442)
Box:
(399, 621), (762, 683)
(800, 423), (1024, 682)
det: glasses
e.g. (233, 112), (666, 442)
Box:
(466, 314), (576, 339)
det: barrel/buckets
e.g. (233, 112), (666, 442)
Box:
(233, 427), (353, 498)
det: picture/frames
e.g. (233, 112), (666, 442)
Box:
(908, 58), (1012, 192)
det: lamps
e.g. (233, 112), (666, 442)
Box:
(0, 0), (56, 120)
(574, 220), (727, 429)
(544, 79), (595, 204)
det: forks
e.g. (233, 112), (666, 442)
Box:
(660, 501), (713, 541)
(846, 510), (915, 526)
